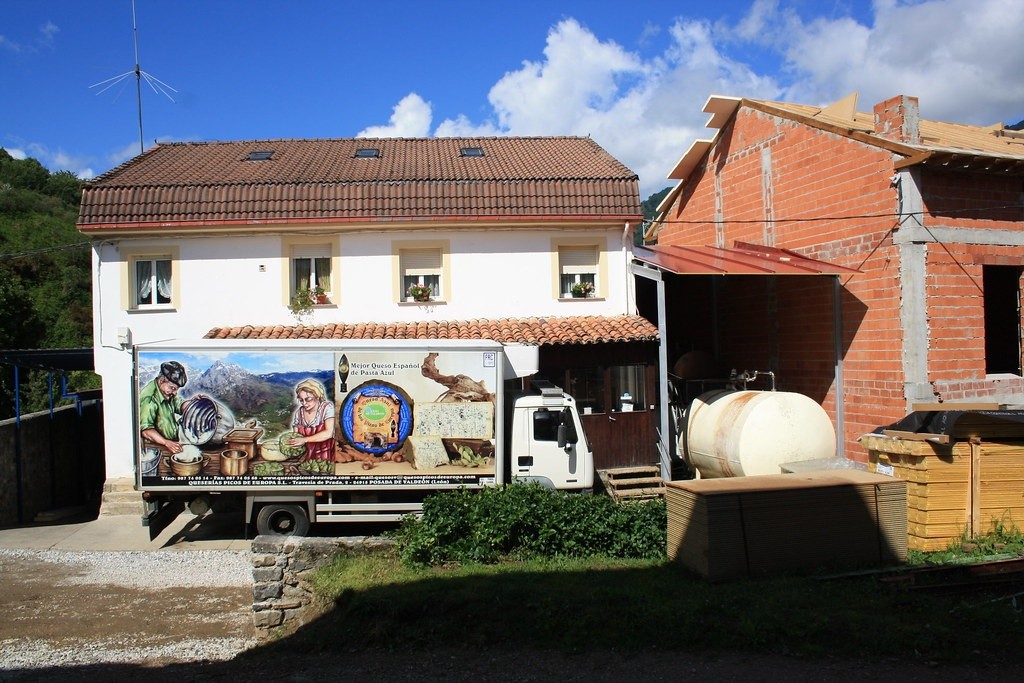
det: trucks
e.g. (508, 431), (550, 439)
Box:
(128, 335), (597, 540)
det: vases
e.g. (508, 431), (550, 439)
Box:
(414, 296), (429, 301)
(317, 295), (326, 303)
(571, 290), (587, 298)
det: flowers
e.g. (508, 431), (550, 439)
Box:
(408, 282), (432, 302)
(306, 286), (324, 300)
(571, 281), (594, 293)
(290, 289), (313, 315)
